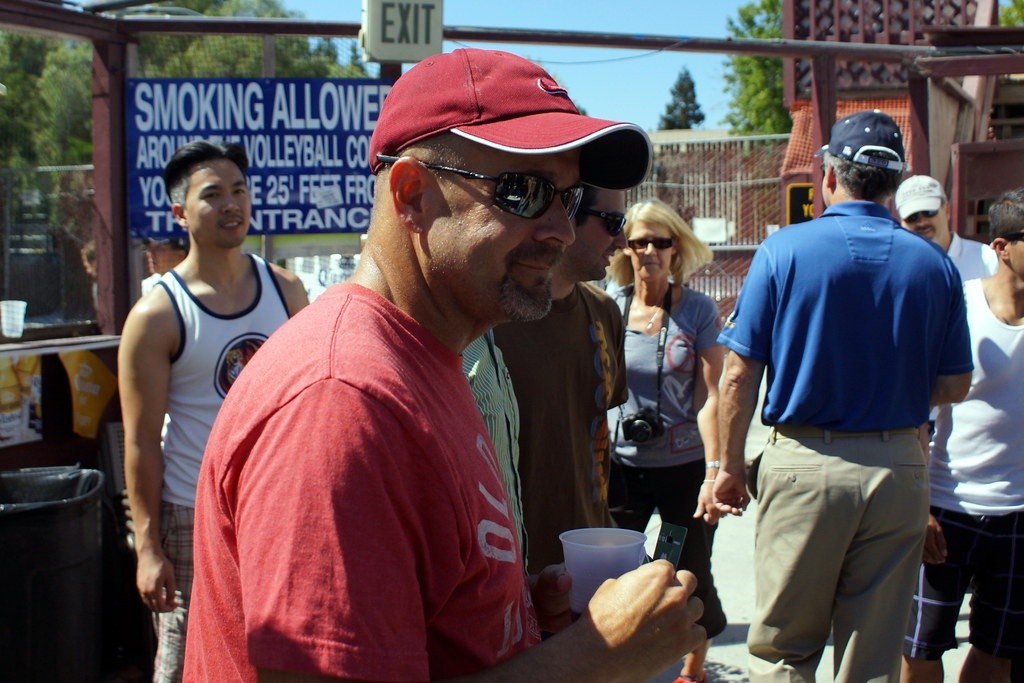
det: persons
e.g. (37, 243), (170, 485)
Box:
(118, 139), (309, 683)
(895, 175), (999, 285)
(603, 196), (727, 683)
(182, 48), (708, 683)
(711, 108), (975, 683)
(490, 182), (629, 574)
(901, 186), (1024, 683)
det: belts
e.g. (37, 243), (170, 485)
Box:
(767, 423), (921, 439)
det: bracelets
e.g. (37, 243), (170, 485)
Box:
(704, 479), (715, 483)
(706, 461), (720, 469)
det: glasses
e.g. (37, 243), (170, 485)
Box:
(375, 150), (584, 221)
(626, 235), (677, 250)
(903, 209), (940, 225)
(576, 204), (627, 237)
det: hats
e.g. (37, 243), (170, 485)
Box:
(368, 48), (654, 191)
(812, 109), (906, 170)
(894, 175), (948, 221)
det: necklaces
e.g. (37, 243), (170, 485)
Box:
(636, 294), (664, 330)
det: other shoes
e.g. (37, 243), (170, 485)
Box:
(672, 670), (707, 683)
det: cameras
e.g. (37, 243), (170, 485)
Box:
(622, 407), (665, 441)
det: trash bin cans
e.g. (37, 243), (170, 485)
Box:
(0, 461), (106, 683)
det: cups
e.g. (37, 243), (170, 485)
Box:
(560, 527), (646, 624)
(0, 300), (28, 339)
